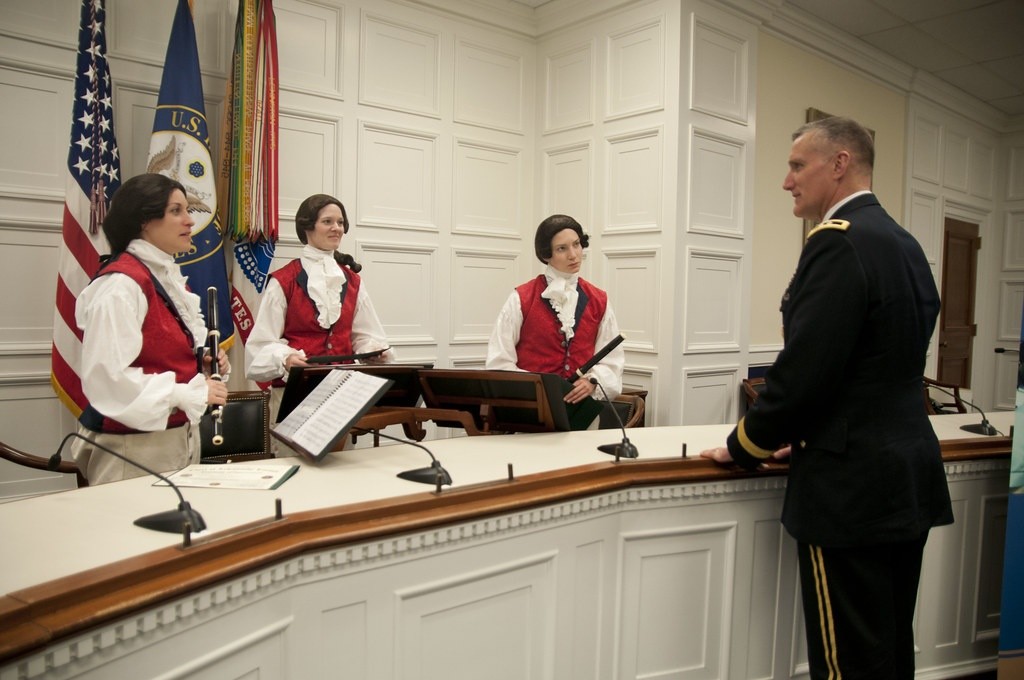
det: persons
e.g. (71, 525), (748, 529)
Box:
(245, 193), (395, 457)
(701, 116), (954, 680)
(71, 173), (231, 485)
(486, 215), (623, 430)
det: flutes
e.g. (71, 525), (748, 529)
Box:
(206, 285), (225, 446)
(567, 332), (626, 384)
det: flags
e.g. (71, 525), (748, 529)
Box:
(52, 0), (123, 419)
(147, 0), (237, 351)
(217, 0), (280, 347)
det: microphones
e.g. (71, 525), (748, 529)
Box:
(588, 378), (639, 460)
(920, 380), (997, 437)
(45, 432), (206, 534)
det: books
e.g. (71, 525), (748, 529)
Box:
(273, 369), (393, 460)
